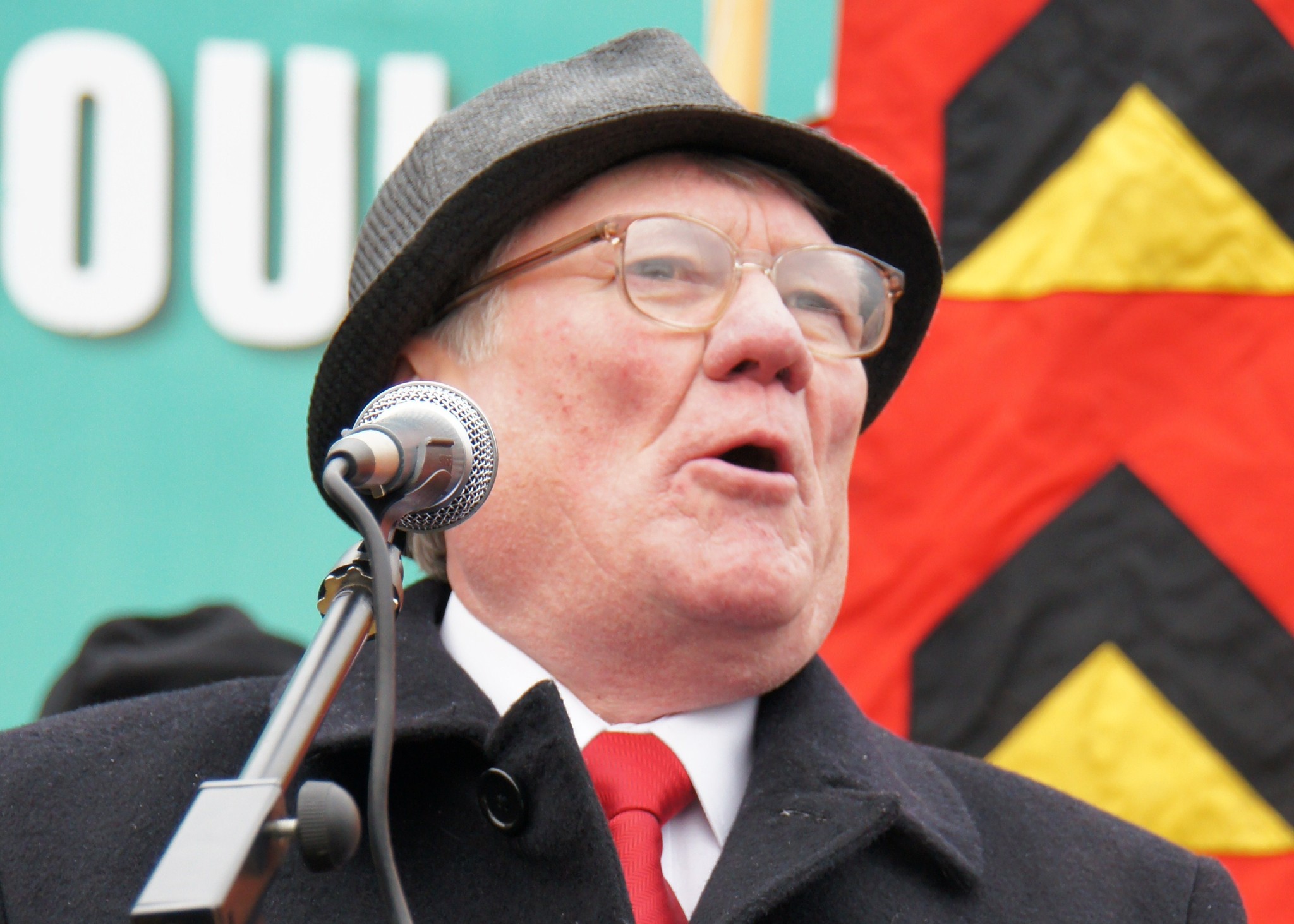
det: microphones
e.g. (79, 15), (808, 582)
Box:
(324, 380), (498, 535)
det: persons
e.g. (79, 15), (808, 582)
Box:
(0, 27), (1247, 924)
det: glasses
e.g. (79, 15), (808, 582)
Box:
(408, 212), (908, 361)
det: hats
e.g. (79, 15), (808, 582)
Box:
(306, 26), (947, 571)
